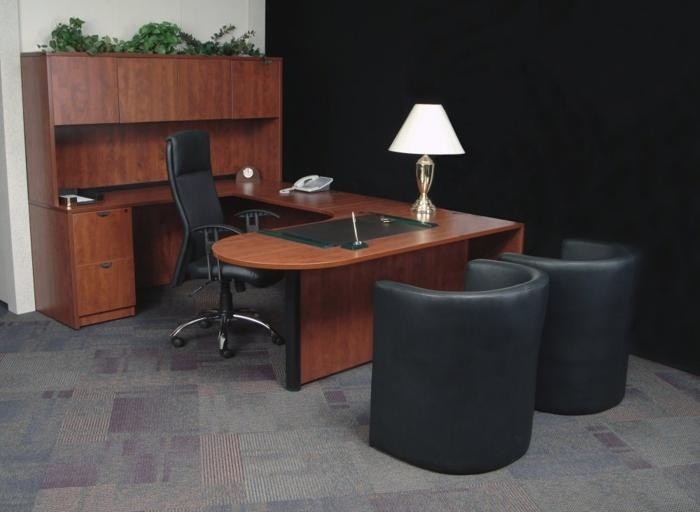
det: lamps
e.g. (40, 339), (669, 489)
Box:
(387, 104), (466, 216)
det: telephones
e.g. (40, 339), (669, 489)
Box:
(289, 174), (333, 194)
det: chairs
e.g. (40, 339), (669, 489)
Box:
(367, 259), (550, 476)
(165, 128), (285, 359)
(502, 236), (639, 415)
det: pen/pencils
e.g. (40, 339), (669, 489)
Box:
(351, 211), (360, 244)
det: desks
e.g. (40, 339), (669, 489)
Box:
(19, 51), (284, 333)
(164, 183), (524, 392)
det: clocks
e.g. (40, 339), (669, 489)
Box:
(236, 165), (261, 185)
